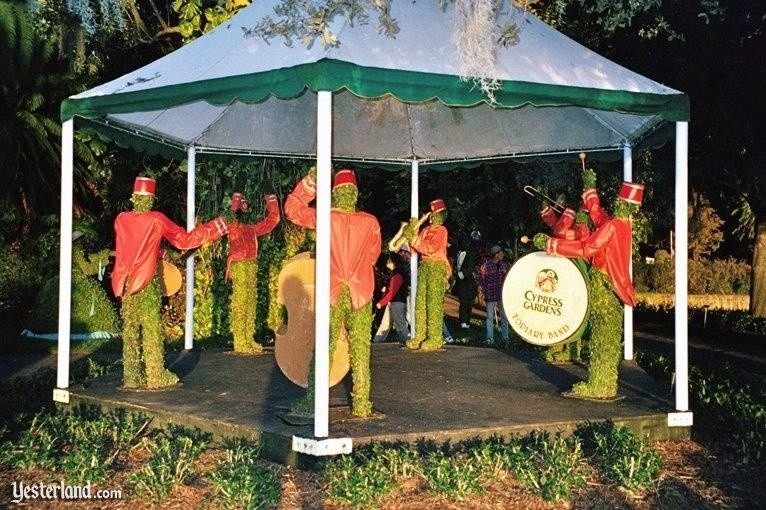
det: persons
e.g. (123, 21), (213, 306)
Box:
(443, 322), (455, 344)
(533, 168), (645, 399)
(373, 254), (409, 343)
(537, 198), (590, 363)
(453, 237), (477, 329)
(403, 199), (452, 350)
(111, 176), (235, 391)
(283, 162), (381, 417)
(223, 192), (281, 354)
(480, 244), (510, 347)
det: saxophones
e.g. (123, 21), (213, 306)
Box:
(388, 212), (432, 252)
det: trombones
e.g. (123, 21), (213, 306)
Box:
(524, 185), (567, 215)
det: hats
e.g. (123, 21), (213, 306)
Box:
(332, 167), (361, 191)
(238, 199), (250, 213)
(616, 179), (647, 205)
(580, 203), (590, 215)
(133, 176), (157, 197)
(490, 244), (503, 256)
(430, 199), (447, 213)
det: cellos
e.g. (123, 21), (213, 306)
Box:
(274, 252), (351, 391)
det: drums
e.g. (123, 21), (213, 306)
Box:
(502, 250), (592, 347)
